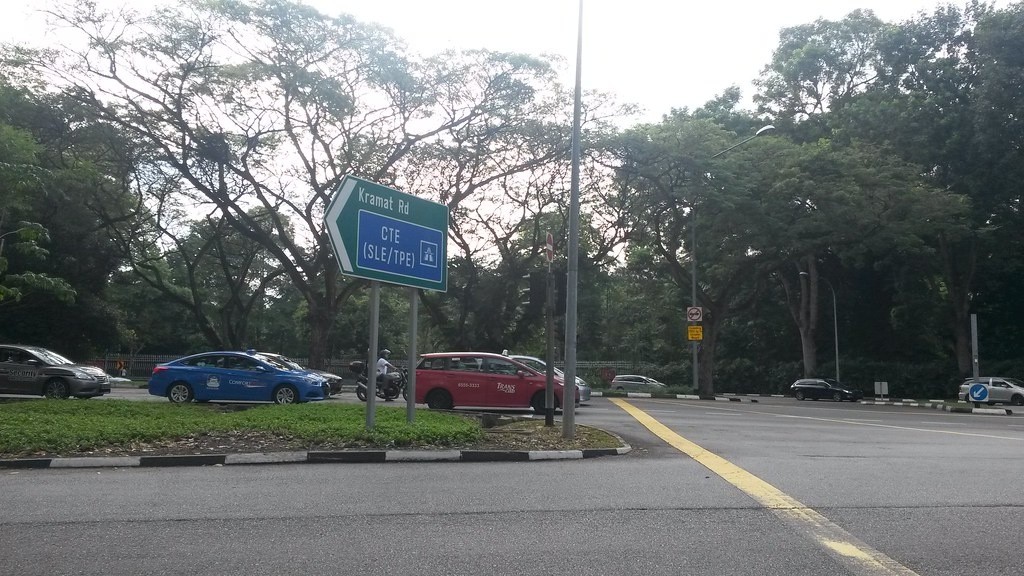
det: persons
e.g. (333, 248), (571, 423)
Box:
(377, 350), (399, 401)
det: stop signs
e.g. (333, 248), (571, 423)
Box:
(545, 230), (554, 262)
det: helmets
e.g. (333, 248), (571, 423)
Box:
(379, 349), (391, 360)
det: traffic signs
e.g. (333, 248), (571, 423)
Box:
(322, 174), (450, 295)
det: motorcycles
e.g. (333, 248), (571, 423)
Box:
(349, 361), (409, 403)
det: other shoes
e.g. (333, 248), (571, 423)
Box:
(379, 391), (384, 395)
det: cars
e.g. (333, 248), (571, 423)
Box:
(416, 352), (581, 415)
(476, 354), (592, 401)
(217, 352), (344, 395)
(790, 378), (863, 401)
(0, 344), (112, 401)
(957, 376), (1024, 408)
(611, 375), (667, 392)
(148, 351), (330, 405)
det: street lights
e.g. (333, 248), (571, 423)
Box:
(799, 270), (839, 380)
(687, 125), (777, 392)
